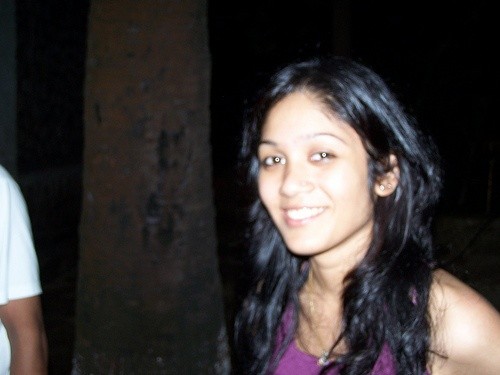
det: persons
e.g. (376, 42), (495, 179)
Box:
(227, 57), (500, 375)
(0, 165), (50, 373)
